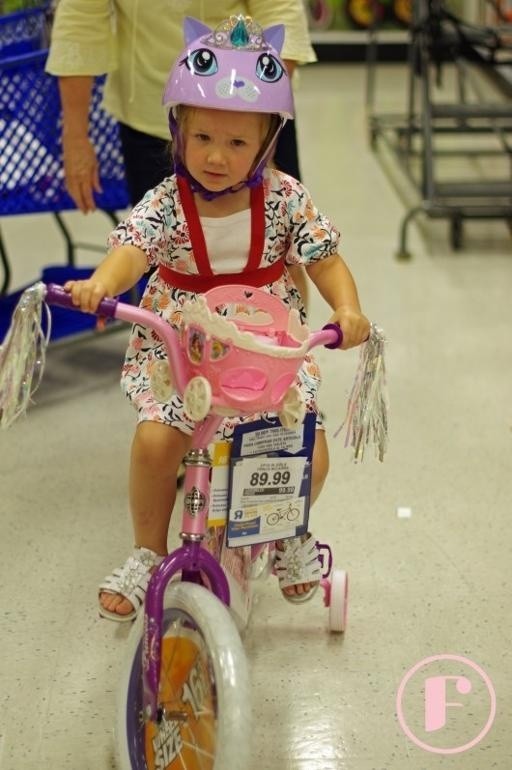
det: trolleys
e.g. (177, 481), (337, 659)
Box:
(0, 6), (148, 344)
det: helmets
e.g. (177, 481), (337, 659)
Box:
(162, 15), (295, 120)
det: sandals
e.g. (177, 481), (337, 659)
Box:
(97, 544), (167, 623)
(275, 524), (322, 605)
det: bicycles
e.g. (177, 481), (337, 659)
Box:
(0, 280), (389, 770)
(266, 503), (300, 525)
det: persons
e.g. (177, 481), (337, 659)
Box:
(62, 17), (371, 624)
(43, 0), (318, 298)
